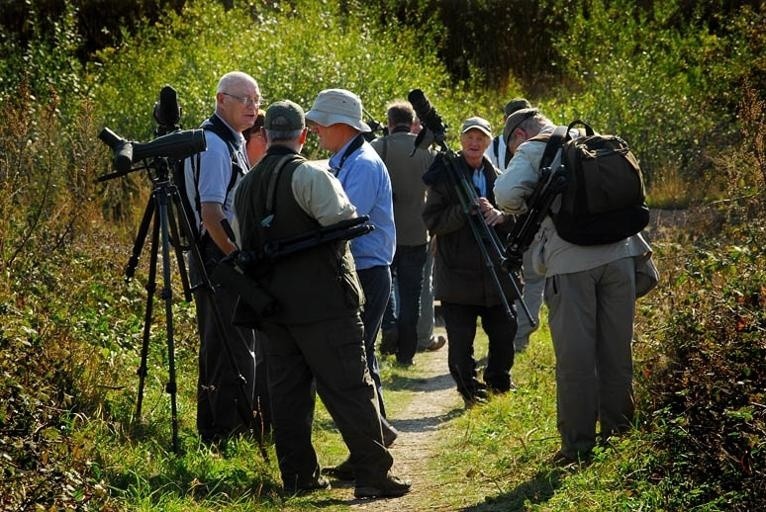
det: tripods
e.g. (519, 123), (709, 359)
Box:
(126, 163), (272, 466)
(500, 164), (566, 274)
(126, 162), (197, 301)
(178, 215), (375, 292)
(433, 144), (536, 329)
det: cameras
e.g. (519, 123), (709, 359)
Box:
(406, 85), (448, 156)
(97, 128), (207, 175)
(152, 86), (183, 134)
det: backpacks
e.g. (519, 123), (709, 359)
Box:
(528, 119), (651, 248)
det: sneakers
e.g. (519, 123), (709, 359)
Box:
(398, 356), (416, 367)
(416, 335), (446, 353)
(320, 459), (356, 478)
(289, 479), (329, 493)
(542, 451), (587, 466)
(354, 471), (413, 497)
(379, 326), (398, 354)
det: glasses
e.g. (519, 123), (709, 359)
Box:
(223, 92), (265, 106)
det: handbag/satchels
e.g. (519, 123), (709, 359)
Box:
(231, 153), (306, 329)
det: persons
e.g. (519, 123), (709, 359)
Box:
(492, 108), (661, 466)
(231, 100), (412, 498)
(422, 115), (525, 405)
(483, 96), (544, 352)
(410, 108), (446, 353)
(241, 107), (268, 170)
(305, 88), (400, 448)
(369, 100), (435, 367)
(182, 69), (260, 441)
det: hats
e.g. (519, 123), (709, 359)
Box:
(504, 97), (532, 115)
(304, 88), (372, 133)
(461, 116), (491, 137)
(502, 107), (539, 147)
(263, 98), (305, 131)
